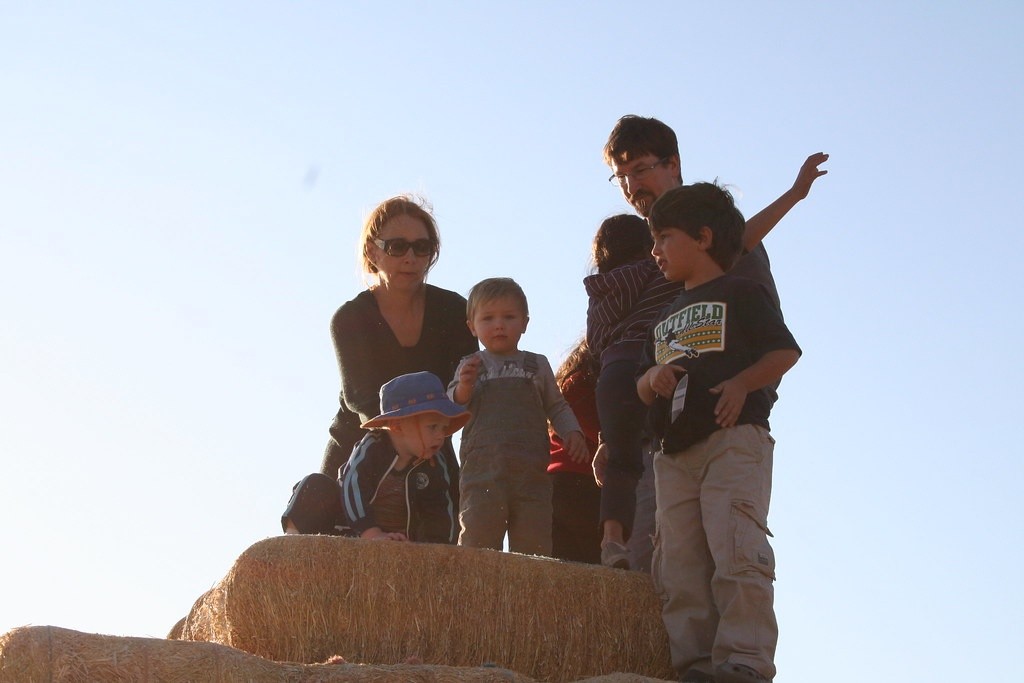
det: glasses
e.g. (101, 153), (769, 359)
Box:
(609, 157), (668, 187)
(374, 237), (434, 256)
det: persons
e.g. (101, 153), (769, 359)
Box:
(281, 117), (829, 682)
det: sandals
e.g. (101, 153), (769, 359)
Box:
(715, 663), (768, 683)
(680, 669), (717, 683)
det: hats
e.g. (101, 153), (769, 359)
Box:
(360, 371), (472, 437)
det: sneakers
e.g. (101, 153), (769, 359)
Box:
(601, 542), (630, 570)
